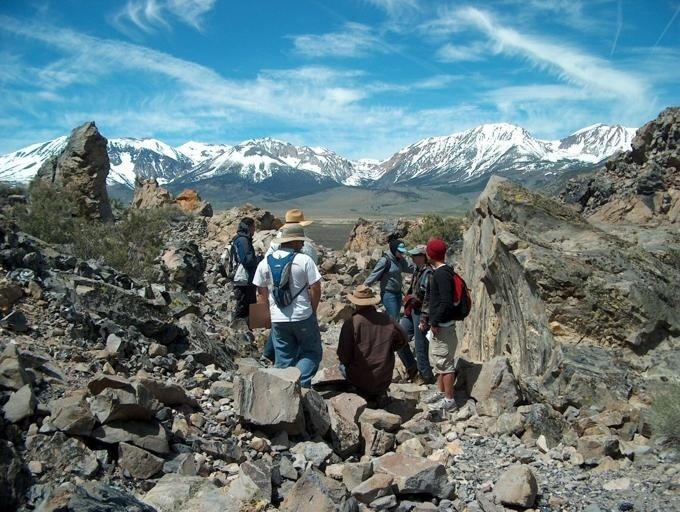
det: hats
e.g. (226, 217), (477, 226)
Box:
(407, 245), (426, 255)
(427, 240), (447, 260)
(280, 209), (314, 230)
(397, 243), (407, 253)
(348, 285), (381, 306)
(271, 225), (306, 244)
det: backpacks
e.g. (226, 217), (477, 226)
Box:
(439, 266), (472, 321)
(219, 235), (248, 281)
(266, 251), (295, 310)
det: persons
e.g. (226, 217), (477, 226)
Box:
(396, 244), (436, 385)
(252, 225), (323, 389)
(336, 285), (418, 395)
(231, 216), (264, 319)
(425, 240), (459, 413)
(255, 208), (318, 368)
(362, 240), (415, 323)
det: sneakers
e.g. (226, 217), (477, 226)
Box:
(424, 392), (442, 403)
(438, 398), (457, 413)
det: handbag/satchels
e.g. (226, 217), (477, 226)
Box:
(403, 295), (414, 317)
